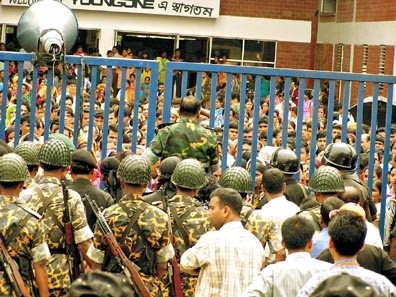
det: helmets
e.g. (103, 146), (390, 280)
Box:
(308, 165), (346, 193)
(0, 153), (28, 182)
(12, 141), (40, 165)
(38, 139), (70, 167)
(116, 154), (152, 185)
(269, 148), (301, 174)
(321, 143), (358, 172)
(171, 158), (209, 189)
(216, 166), (251, 193)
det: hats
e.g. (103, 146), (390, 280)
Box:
(71, 149), (98, 169)
(68, 270), (135, 297)
(77, 135), (96, 151)
(329, 202), (365, 219)
(307, 272), (379, 297)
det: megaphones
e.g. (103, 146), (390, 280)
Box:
(16, 0), (79, 64)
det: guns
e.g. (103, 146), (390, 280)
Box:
(84, 194), (152, 297)
(0, 237), (34, 297)
(60, 179), (81, 284)
(166, 206), (184, 297)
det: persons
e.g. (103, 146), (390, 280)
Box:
(245, 216), (334, 297)
(0, 41), (396, 266)
(86, 154), (175, 297)
(19, 138), (93, 297)
(295, 210), (396, 297)
(179, 188), (265, 297)
(70, 270), (134, 297)
(0, 154), (51, 297)
(316, 202), (395, 287)
(157, 159), (216, 297)
